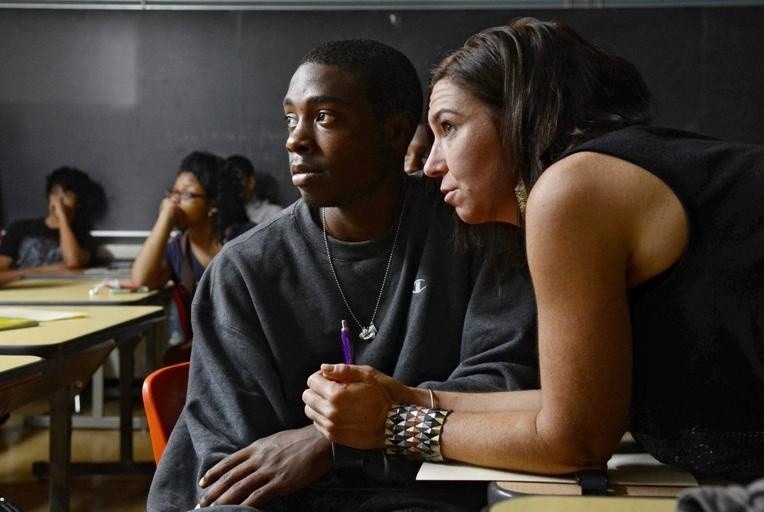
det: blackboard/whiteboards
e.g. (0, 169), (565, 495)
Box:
(0, 3), (763, 238)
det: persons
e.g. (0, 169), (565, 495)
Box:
(0, 165), (104, 283)
(143, 37), (539, 512)
(227, 155), (285, 225)
(298, 16), (763, 488)
(129, 152), (258, 348)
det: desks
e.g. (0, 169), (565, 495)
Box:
(2, 302), (165, 508)
(0, 350), (45, 387)
(1, 263), (174, 479)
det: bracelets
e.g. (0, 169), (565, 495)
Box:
(427, 386), (435, 408)
(382, 401), (452, 466)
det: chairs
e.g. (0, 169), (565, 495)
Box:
(141, 360), (189, 469)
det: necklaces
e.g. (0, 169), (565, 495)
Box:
(321, 190), (405, 342)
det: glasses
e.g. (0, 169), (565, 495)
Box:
(163, 187), (214, 202)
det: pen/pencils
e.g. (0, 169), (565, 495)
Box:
(341, 319), (353, 363)
